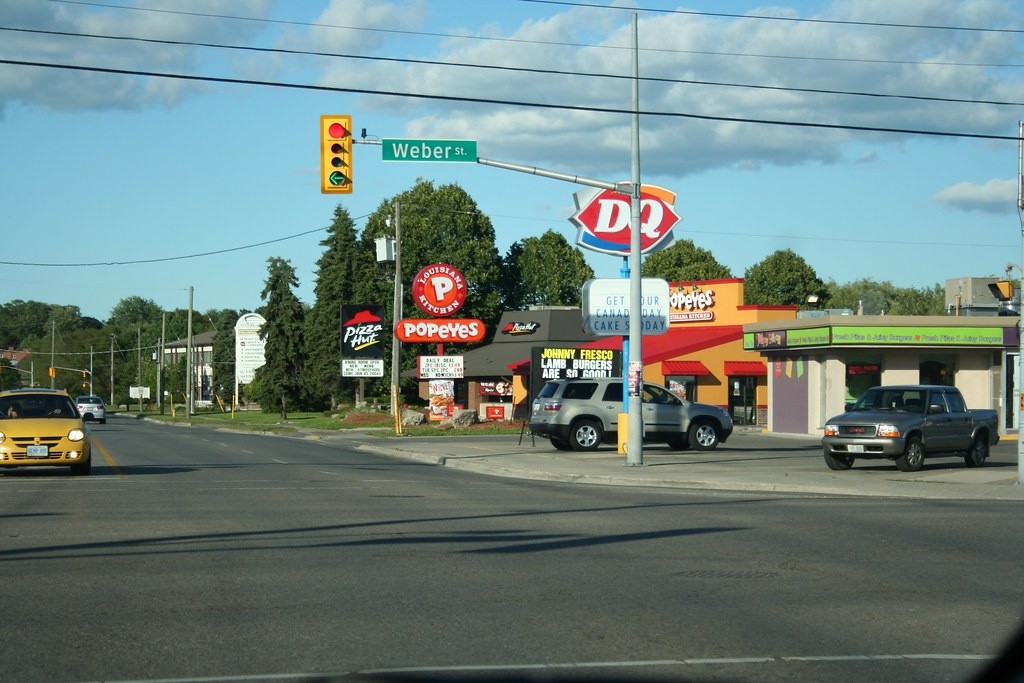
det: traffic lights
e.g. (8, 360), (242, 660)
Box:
(987, 282), (1009, 300)
(319, 114), (353, 195)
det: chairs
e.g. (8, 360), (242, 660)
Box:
(889, 396), (904, 408)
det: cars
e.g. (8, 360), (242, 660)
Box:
(75, 396), (107, 424)
(0, 387), (92, 471)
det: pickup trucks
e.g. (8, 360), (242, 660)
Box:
(821, 384), (999, 472)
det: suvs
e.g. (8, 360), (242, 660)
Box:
(530, 376), (733, 451)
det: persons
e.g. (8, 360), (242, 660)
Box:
(9, 402), (23, 418)
(43, 398), (69, 415)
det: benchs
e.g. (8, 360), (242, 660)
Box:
(905, 398), (925, 407)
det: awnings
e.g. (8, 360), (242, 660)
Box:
(508, 325), (744, 372)
(724, 361), (767, 376)
(661, 360), (711, 376)
